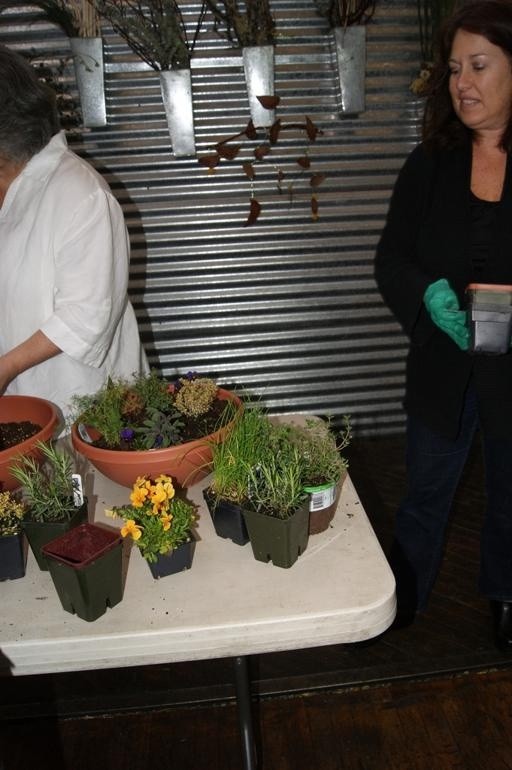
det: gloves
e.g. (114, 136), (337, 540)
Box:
(422, 278), (473, 353)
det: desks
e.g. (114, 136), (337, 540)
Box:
(0, 414), (413, 769)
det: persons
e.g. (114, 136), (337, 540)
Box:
(368, 4), (510, 655)
(1, 45), (159, 437)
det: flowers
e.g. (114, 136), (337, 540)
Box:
(70, 375), (217, 449)
(0, 488), (24, 536)
(101, 462), (196, 563)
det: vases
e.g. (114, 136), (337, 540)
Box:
(41, 522), (124, 623)
(460, 283), (512, 357)
(0, 537), (26, 581)
(139, 528), (200, 581)
(71, 385), (246, 490)
(0, 395), (59, 494)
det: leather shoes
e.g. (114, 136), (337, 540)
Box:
(490, 596), (512, 657)
(389, 599), (417, 631)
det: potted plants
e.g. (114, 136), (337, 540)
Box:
(93, 1), (206, 156)
(251, 434), (313, 568)
(208, 0), (277, 128)
(37, 1), (107, 127)
(15, 443), (81, 570)
(285, 420), (347, 535)
(205, 421), (245, 549)
(318, 3), (377, 116)
